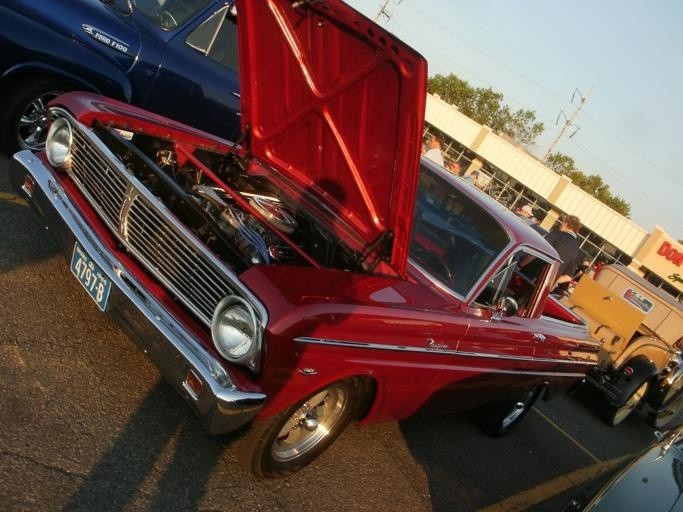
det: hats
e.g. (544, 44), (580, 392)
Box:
(522, 205), (534, 216)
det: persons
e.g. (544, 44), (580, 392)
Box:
(573, 258), (591, 282)
(447, 159), (462, 177)
(463, 171), (480, 184)
(422, 133), (444, 167)
(522, 214), (582, 293)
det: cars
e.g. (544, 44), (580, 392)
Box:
(7, 0), (604, 480)
(0, 0), (242, 159)
(546, 263), (683, 512)
